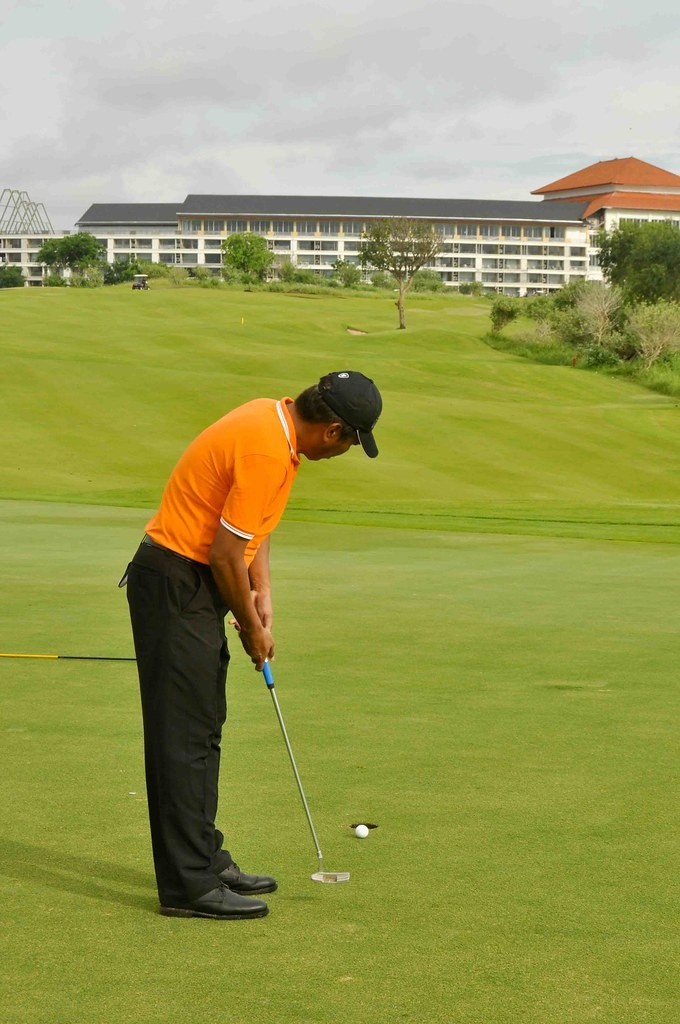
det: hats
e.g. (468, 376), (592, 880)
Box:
(320, 370), (382, 458)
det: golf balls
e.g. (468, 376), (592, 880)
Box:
(354, 825), (369, 839)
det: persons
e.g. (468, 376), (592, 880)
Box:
(142, 277), (147, 288)
(118, 370), (382, 920)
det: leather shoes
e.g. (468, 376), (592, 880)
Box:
(160, 882), (269, 919)
(217, 862), (278, 894)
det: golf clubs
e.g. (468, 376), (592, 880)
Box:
(264, 658), (351, 885)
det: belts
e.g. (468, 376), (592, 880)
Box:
(142, 534), (199, 566)
